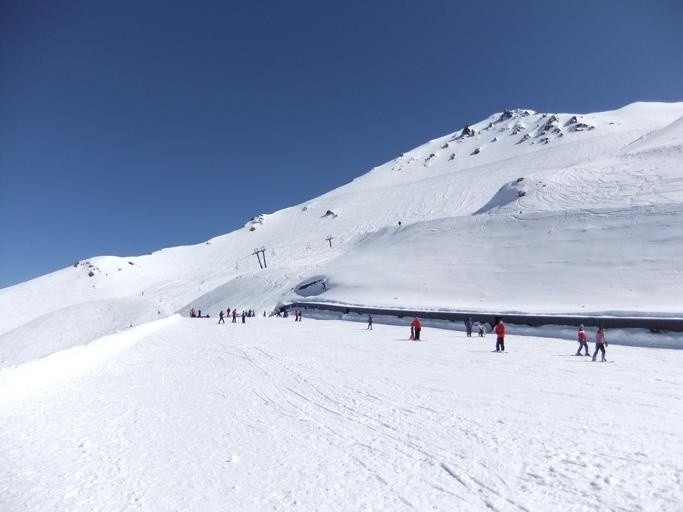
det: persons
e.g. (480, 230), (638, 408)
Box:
(464, 316), (474, 337)
(411, 316), (421, 340)
(591, 325), (608, 363)
(367, 314), (373, 331)
(574, 323), (591, 357)
(494, 320), (505, 352)
(188, 307), (303, 324)
